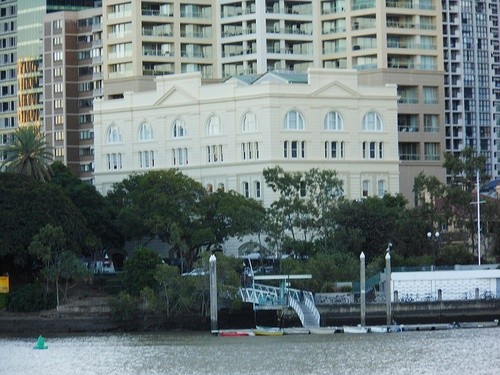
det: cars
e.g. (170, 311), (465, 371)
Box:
(182, 267), (210, 277)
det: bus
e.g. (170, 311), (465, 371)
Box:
(233, 254), (309, 275)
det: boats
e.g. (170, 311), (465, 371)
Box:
(218, 319), (498, 336)
